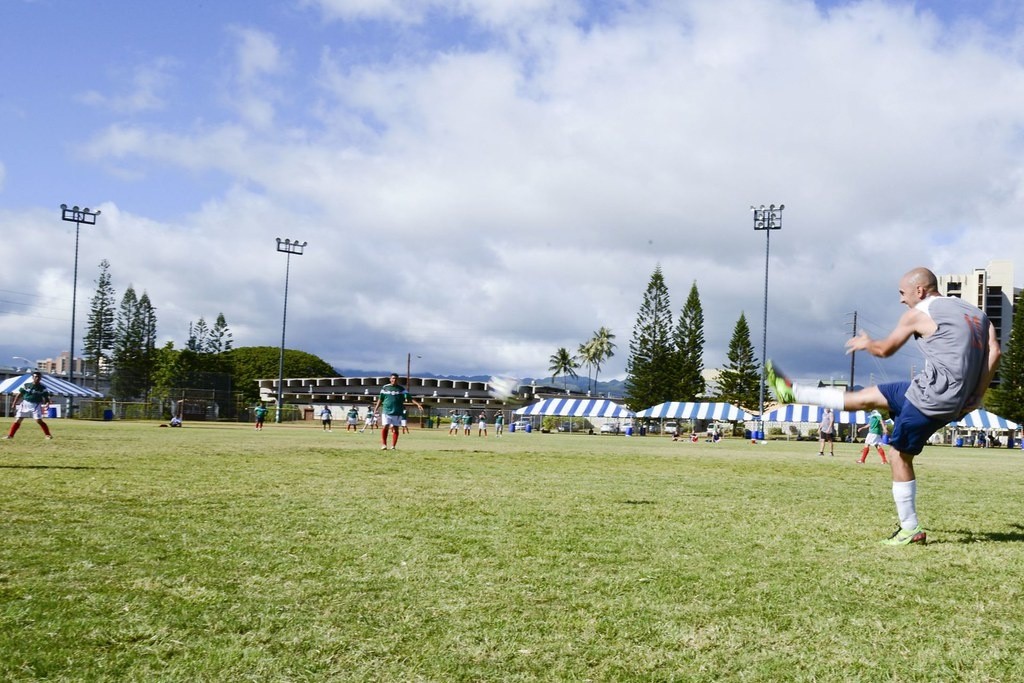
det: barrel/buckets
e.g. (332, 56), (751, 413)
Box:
(956, 438), (963, 446)
(882, 434), (888, 443)
(508, 423), (516, 432)
(525, 424), (532, 433)
(104, 410), (113, 420)
(48, 408), (56, 418)
(625, 428), (632, 437)
(640, 428), (646, 435)
(744, 431), (764, 440)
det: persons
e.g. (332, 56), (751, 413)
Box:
(854, 410), (889, 465)
(449, 410), (473, 436)
(359, 405), (379, 434)
(969, 430), (984, 448)
(320, 404), (333, 432)
(254, 402), (268, 431)
(767, 267), (1000, 547)
(797, 434), (803, 440)
(672, 421), (680, 442)
(616, 422), (620, 435)
(436, 415), (440, 428)
(478, 410), (487, 437)
(688, 430), (698, 441)
(493, 410), (505, 438)
(401, 407), (409, 434)
(2, 372), (53, 440)
(589, 428), (593, 435)
(374, 373), (423, 450)
(816, 407), (835, 457)
(810, 433), (814, 439)
(170, 414), (182, 427)
(345, 404), (360, 433)
(712, 421), (721, 443)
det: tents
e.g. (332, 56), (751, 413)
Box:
(0, 373), (104, 417)
(944, 408), (1022, 447)
(511, 398), (894, 441)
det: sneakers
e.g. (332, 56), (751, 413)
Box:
(381, 444), (387, 450)
(46, 434), (52, 439)
(829, 452), (834, 456)
(1, 435), (13, 440)
(390, 446), (396, 450)
(881, 461), (888, 465)
(881, 524), (927, 544)
(766, 360), (794, 404)
(856, 459), (865, 464)
(818, 452), (824, 455)
